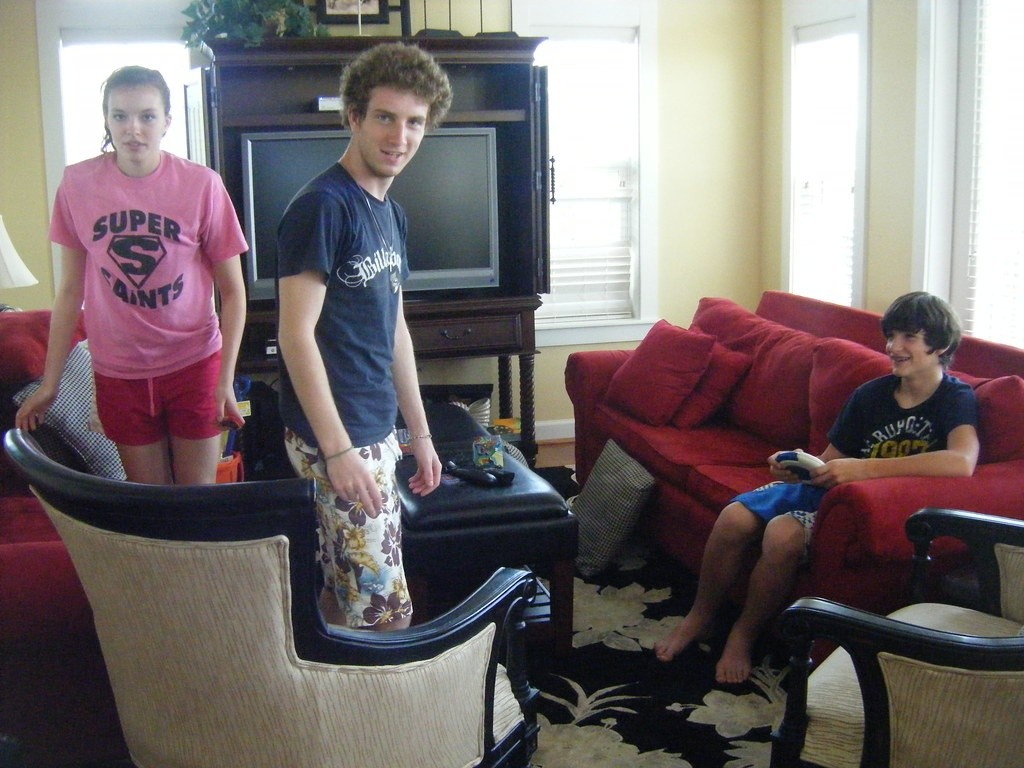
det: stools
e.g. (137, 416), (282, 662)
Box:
(390, 403), (578, 647)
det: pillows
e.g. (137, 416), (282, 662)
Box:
(564, 439), (656, 580)
(690, 296), (775, 406)
(732, 316), (821, 448)
(12, 339), (128, 485)
(809, 334), (892, 454)
(607, 318), (716, 426)
(673, 325), (752, 430)
(950, 370), (1023, 462)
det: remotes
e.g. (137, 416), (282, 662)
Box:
(452, 466), (515, 485)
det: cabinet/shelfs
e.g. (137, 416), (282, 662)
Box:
(200, 32), (547, 465)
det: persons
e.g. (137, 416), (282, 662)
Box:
(656, 292), (980, 683)
(274, 42), (453, 631)
(15, 65), (249, 484)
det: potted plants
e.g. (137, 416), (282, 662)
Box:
(183, 0), (328, 63)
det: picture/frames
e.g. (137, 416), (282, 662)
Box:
(317, 0), (391, 25)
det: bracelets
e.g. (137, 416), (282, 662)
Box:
(410, 434), (432, 441)
(323, 446), (354, 461)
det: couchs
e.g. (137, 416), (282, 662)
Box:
(1, 310), (85, 390)
(0, 495), (93, 641)
(564, 288), (1023, 663)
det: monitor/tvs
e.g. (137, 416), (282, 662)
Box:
(241, 127), (500, 302)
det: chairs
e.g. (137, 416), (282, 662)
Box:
(0, 427), (540, 768)
(769, 508), (1024, 768)
